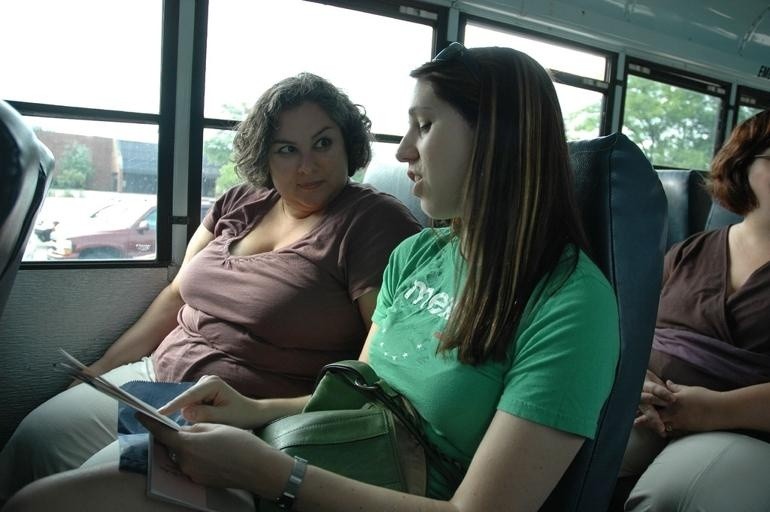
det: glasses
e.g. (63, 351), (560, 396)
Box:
(432, 42), (482, 84)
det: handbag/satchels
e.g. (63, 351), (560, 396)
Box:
(249, 359), (467, 511)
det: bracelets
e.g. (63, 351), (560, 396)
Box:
(276, 453), (308, 509)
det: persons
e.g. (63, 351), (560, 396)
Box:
(606, 108), (770, 511)
(0, 71), (423, 510)
(5, 44), (624, 512)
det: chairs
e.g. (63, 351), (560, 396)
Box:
(358, 134), (746, 509)
(1, 101), (57, 337)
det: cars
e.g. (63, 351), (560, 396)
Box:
(32, 192), (213, 260)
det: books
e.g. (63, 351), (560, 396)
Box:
(147, 435), (209, 511)
(46, 347), (182, 434)
